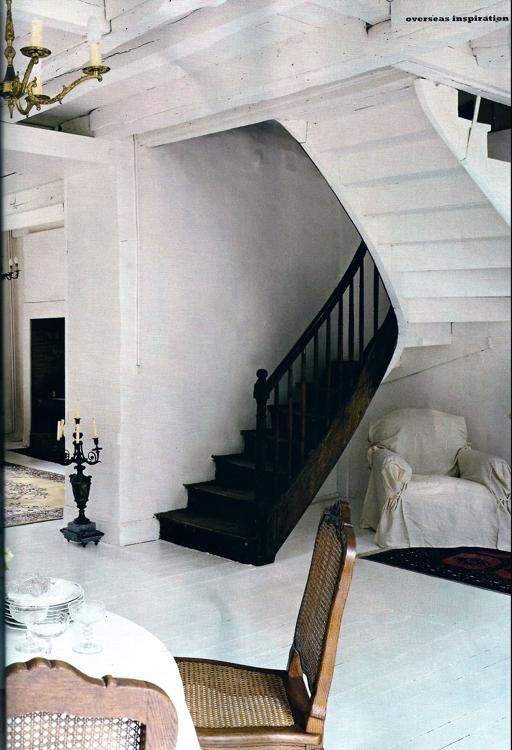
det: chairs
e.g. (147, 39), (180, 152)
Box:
(368, 408), (511, 555)
(176, 500), (358, 748)
(5, 656), (179, 750)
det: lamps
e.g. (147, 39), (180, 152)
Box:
(0, 1), (111, 121)
(1, 255), (22, 281)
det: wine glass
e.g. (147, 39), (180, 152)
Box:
(9, 601), (51, 653)
(68, 601), (108, 657)
(24, 610), (69, 661)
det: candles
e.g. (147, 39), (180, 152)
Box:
(56, 398), (99, 445)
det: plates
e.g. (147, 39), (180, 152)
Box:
(3, 576), (86, 632)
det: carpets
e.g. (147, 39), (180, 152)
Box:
(358, 545), (510, 597)
(3, 460), (65, 528)
